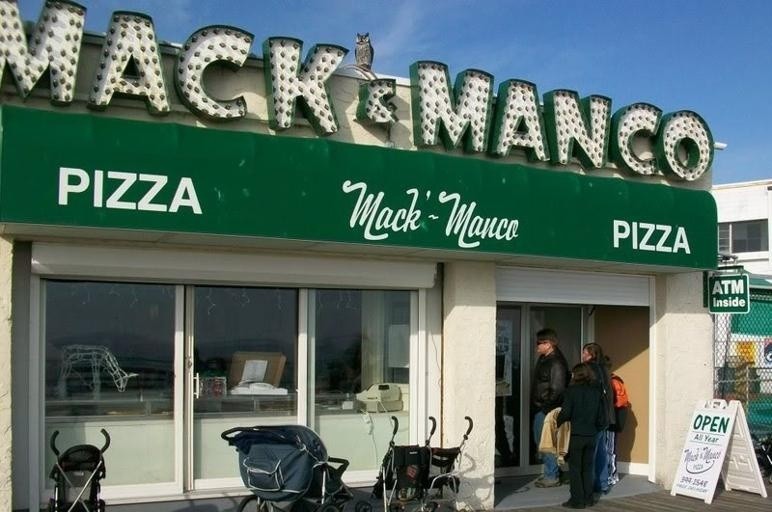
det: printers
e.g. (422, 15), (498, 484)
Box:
(356, 384), (403, 412)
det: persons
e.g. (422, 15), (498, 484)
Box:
(531, 327), (570, 487)
(602, 354), (627, 486)
(581, 342), (615, 494)
(558, 361), (609, 510)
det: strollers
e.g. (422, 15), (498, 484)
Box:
(355, 417), (473, 512)
(47, 429), (110, 512)
(221, 425), (354, 512)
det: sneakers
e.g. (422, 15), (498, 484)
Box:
(562, 501), (593, 508)
(534, 477), (560, 489)
